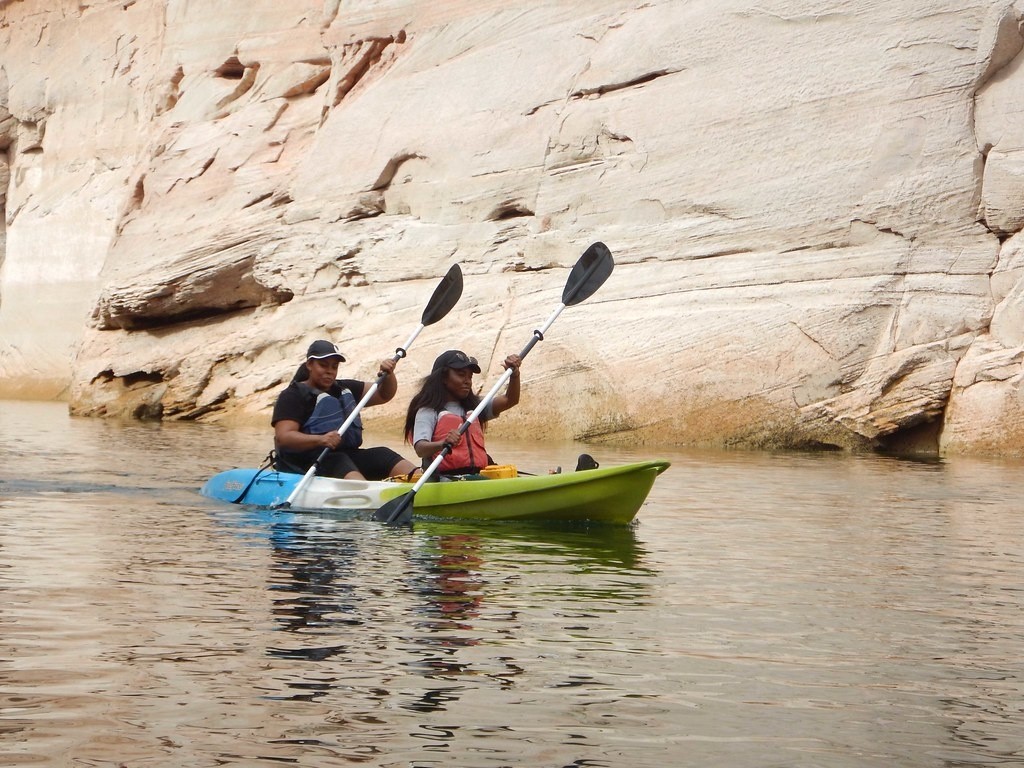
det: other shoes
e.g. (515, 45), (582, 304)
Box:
(548, 467), (562, 474)
(575, 453), (599, 472)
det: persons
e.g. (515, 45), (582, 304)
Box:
(271, 339), (422, 483)
(403, 350), (600, 482)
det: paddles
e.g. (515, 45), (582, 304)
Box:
(371, 240), (616, 522)
(280, 261), (465, 508)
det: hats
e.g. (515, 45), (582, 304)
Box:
(433, 350), (481, 373)
(306, 340), (346, 362)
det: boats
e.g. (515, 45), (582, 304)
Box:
(197, 457), (673, 530)
